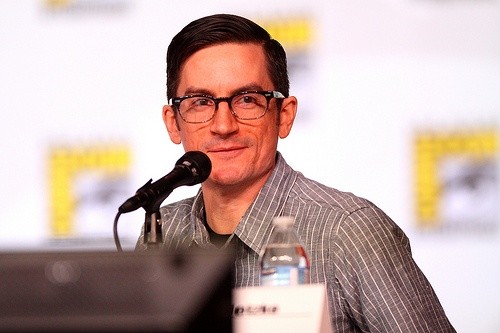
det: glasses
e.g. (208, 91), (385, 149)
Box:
(169, 91), (285, 124)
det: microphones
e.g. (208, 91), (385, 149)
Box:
(119, 151), (212, 213)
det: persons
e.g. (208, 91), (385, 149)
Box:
(135, 13), (457, 333)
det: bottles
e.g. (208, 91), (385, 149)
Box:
(260, 215), (310, 285)
